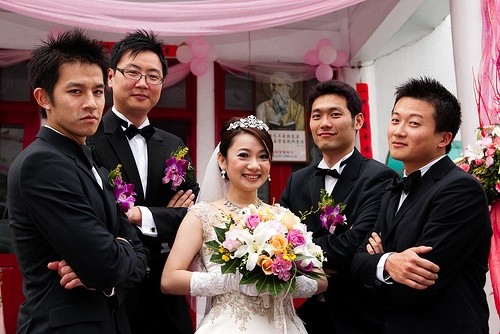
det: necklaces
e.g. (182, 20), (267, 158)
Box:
(223, 195), (261, 215)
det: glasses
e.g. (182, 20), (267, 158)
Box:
(115, 68), (165, 85)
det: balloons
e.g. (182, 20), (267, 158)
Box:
(48, 26), (63, 42)
(305, 38), (347, 82)
(176, 35), (215, 76)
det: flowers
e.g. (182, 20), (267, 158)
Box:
(204, 204), (331, 296)
(453, 127), (500, 196)
(318, 189), (347, 234)
(108, 164), (137, 209)
(162, 146), (195, 190)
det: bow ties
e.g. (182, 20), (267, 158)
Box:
(80, 140), (102, 169)
(384, 174), (413, 195)
(125, 124), (156, 142)
(313, 165), (341, 179)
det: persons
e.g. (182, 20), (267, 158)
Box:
(280, 79), (399, 334)
(159, 114), (328, 334)
(86, 29), (200, 334)
(351, 76), (491, 334)
(8, 29), (146, 334)
